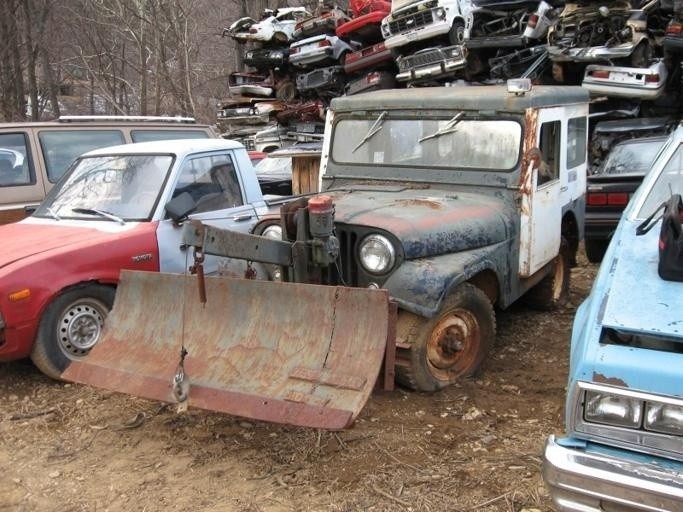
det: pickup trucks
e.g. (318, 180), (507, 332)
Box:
(0, 138), (297, 385)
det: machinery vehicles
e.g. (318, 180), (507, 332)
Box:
(239, 79), (595, 394)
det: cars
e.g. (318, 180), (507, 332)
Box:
(581, 134), (675, 264)
(542, 120), (683, 512)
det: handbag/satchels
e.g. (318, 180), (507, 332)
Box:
(636, 194), (683, 283)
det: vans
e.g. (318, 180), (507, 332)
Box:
(0, 115), (218, 237)
